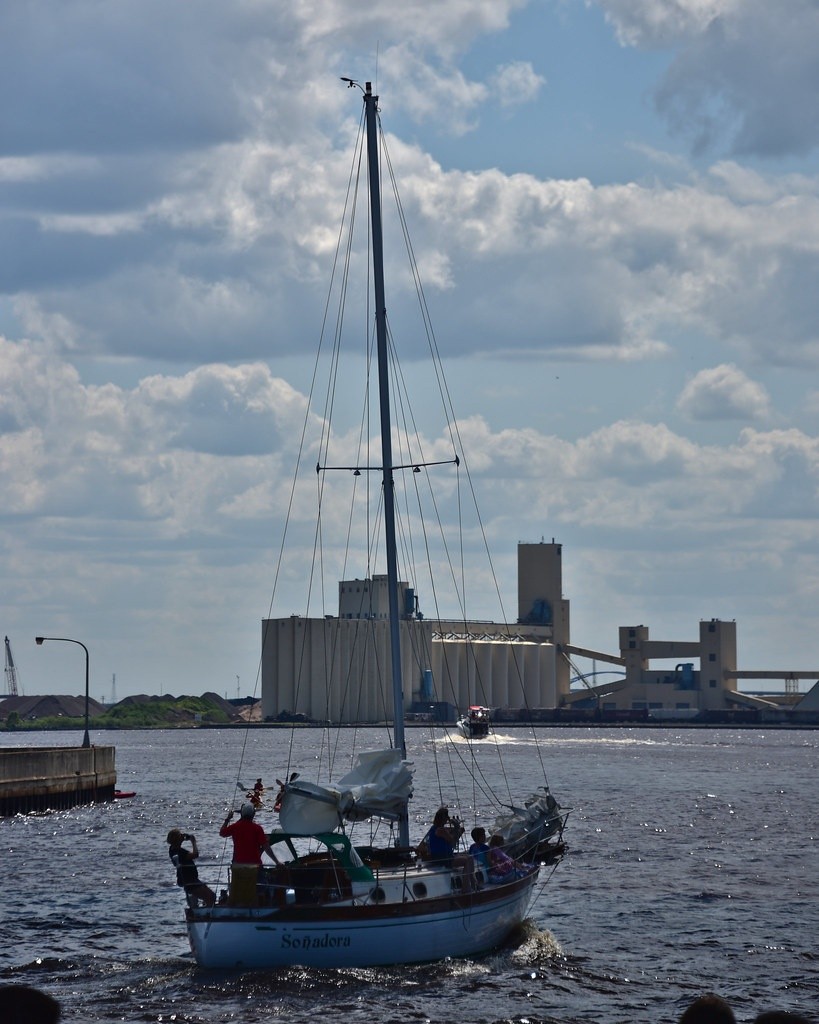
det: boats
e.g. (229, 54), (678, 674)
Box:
(458, 704), (491, 740)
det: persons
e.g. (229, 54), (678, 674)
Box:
(252, 777), (264, 794)
(470, 711), (478, 719)
(488, 832), (532, 876)
(220, 803), (285, 904)
(466, 826), (492, 863)
(0, 981), (64, 1024)
(246, 790), (263, 807)
(166, 827), (217, 907)
(427, 806), (483, 892)
(676, 994), (738, 1023)
(273, 801), (281, 811)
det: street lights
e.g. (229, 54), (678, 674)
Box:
(35, 637), (91, 748)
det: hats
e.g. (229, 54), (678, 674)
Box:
(232, 803), (256, 815)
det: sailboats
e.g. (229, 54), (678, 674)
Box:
(177, 75), (568, 972)
